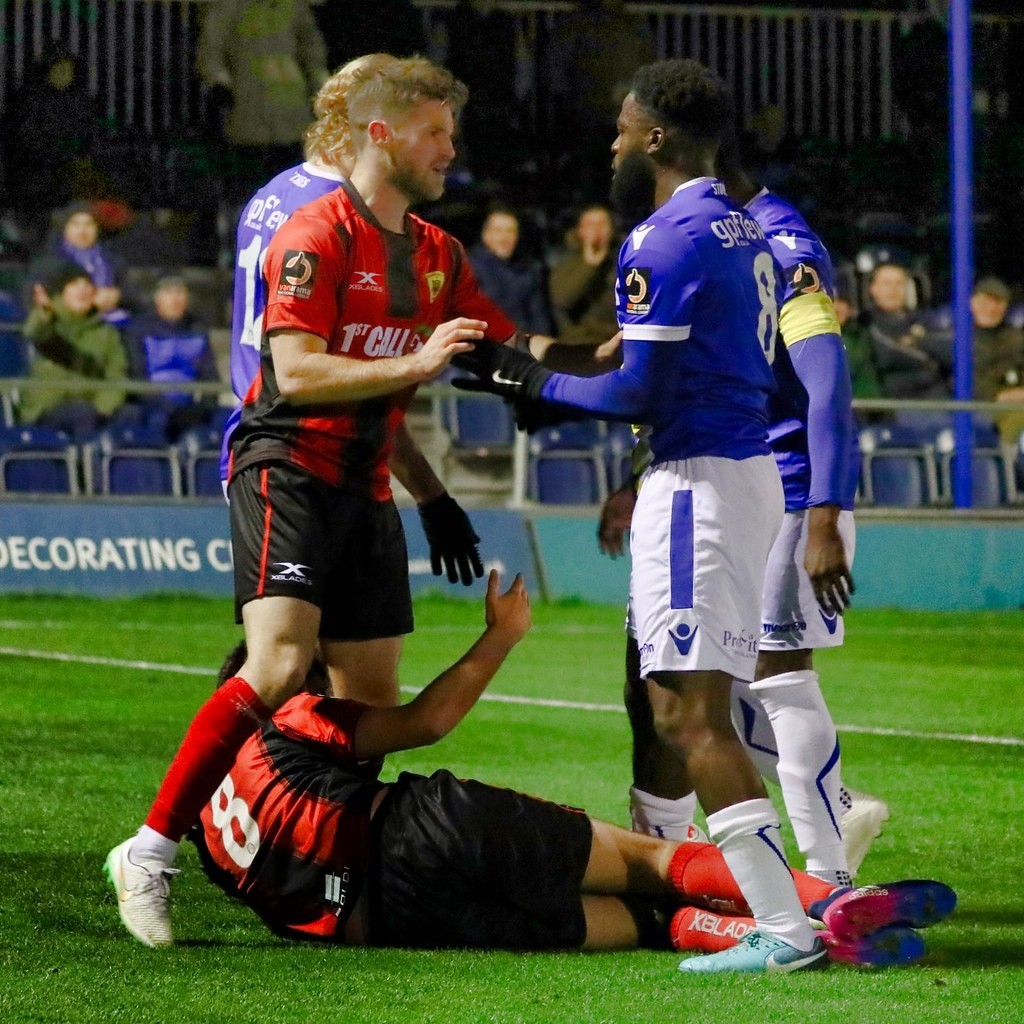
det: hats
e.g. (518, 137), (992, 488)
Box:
(975, 276), (1011, 300)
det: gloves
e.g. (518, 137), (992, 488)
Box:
(450, 336), (555, 404)
(512, 400), (585, 434)
(416, 491), (484, 587)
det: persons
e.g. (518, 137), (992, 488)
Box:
(23, 202), (146, 330)
(0, 41), (106, 236)
(103, 58), (625, 948)
(17, 268), (148, 443)
(196, 0), (332, 186)
(864, 261), (953, 402)
(969, 276), (1024, 403)
(216, 53), (487, 585)
(190, 567), (957, 969)
(114, 270), (222, 447)
(547, 206), (629, 345)
(448, 58), (831, 973)
(469, 210), (551, 336)
(596, 117), (896, 888)
(834, 291), (882, 400)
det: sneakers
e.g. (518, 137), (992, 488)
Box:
(677, 931), (829, 973)
(102, 837), (182, 951)
(813, 926), (926, 966)
(808, 879), (957, 940)
(840, 789), (889, 877)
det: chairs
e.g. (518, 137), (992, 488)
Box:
(1, 261), (1019, 507)
